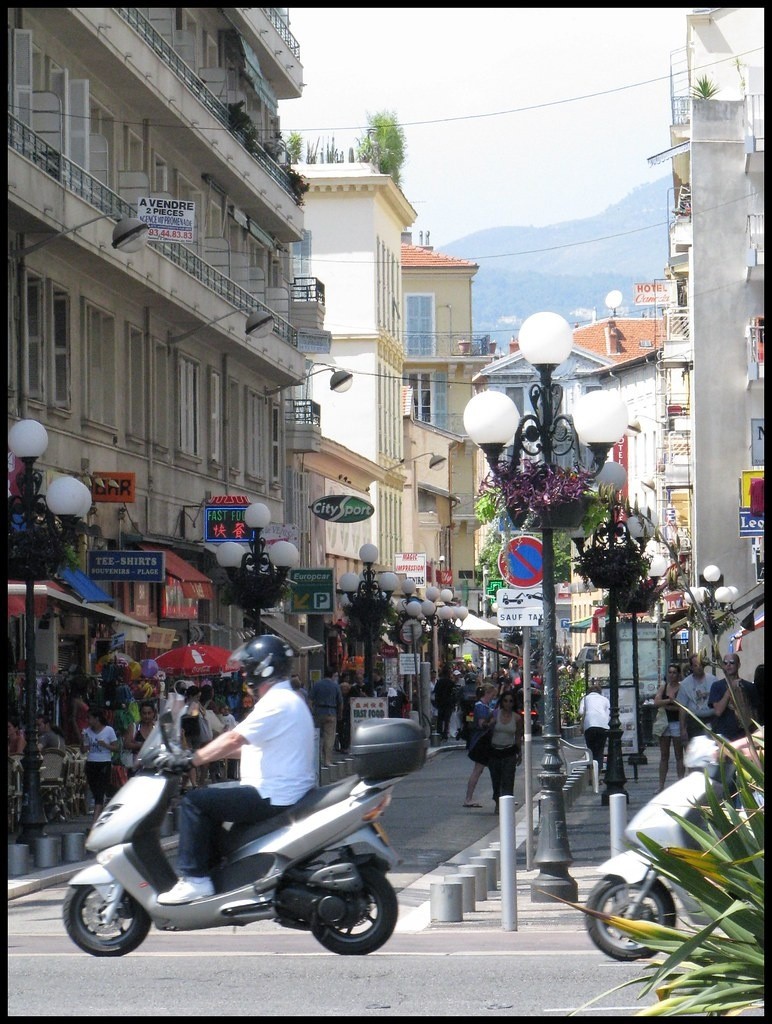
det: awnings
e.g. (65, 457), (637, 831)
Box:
(8, 519), (146, 630)
(143, 544), (214, 599)
(256, 615), (324, 655)
(422, 612), (501, 634)
(340, 619), (398, 657)
(713, 580), (764, 654)
(569, 616), (610, 633)
(469, 635), (520, 661)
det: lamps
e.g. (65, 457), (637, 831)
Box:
(11, 213), (148, 266)
(167, 305), (274, 354)
(266, 367), (353, 397)
(385, 452), (448, 473)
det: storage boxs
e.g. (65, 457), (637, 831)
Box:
(351, 717), (427, 779)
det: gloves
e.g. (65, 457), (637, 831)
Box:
(152, 751), (195, 774)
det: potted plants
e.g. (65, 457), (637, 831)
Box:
(472, 458), (662, 615)
(558, 673), (585, 738)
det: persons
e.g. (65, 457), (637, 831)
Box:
(290, 670), (384, 767)
(578, 686), (610, 785)
(67, 678), (89, 744)
(431, 666), (542, 740)
(654, 654), (763, 794)
(463, 683), (523, 815)
(80, 711), (120, 830)
(124, 683), (237, 790)
(157, 634), (318, 903)
(8, 716), (65, 821)
(712, 726), (764, 766)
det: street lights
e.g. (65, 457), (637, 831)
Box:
(568, 462), (659, 807)
(396, 577), (437, 720)
(457, 309), (631, 903)
(6, 418), (94, 855)
(422, 586), (455, 667)
(683, 564), (741, 676)
(619, 555), (669, 766)
(338, 543), (401, 697)
(438, 589), (470, 668)
(214, 502), (300, 636)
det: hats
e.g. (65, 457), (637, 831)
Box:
(453, 670), (461, 675)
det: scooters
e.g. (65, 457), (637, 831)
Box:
(59, 709), (431, 958)
(577, 724), (764, 959)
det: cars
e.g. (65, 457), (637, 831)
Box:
(555, 646), (598, 673)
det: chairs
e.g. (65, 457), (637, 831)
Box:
(6, 746), (88, 831)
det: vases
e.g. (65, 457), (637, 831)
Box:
(458, 341), (472, 352)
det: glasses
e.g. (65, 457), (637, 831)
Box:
(668, 663), (680, 666)
(723, 660), (738, 665)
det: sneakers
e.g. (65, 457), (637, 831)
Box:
(157, 877), (216, 904)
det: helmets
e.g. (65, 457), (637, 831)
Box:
(237, 634), (293, 689)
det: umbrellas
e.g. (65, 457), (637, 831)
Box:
(153, 641), (244, 685)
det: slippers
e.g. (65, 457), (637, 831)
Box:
(463, 802), (482, 807)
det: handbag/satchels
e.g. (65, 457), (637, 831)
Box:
(652, 706), (669, 737)
(467, 737), (492, 767)
